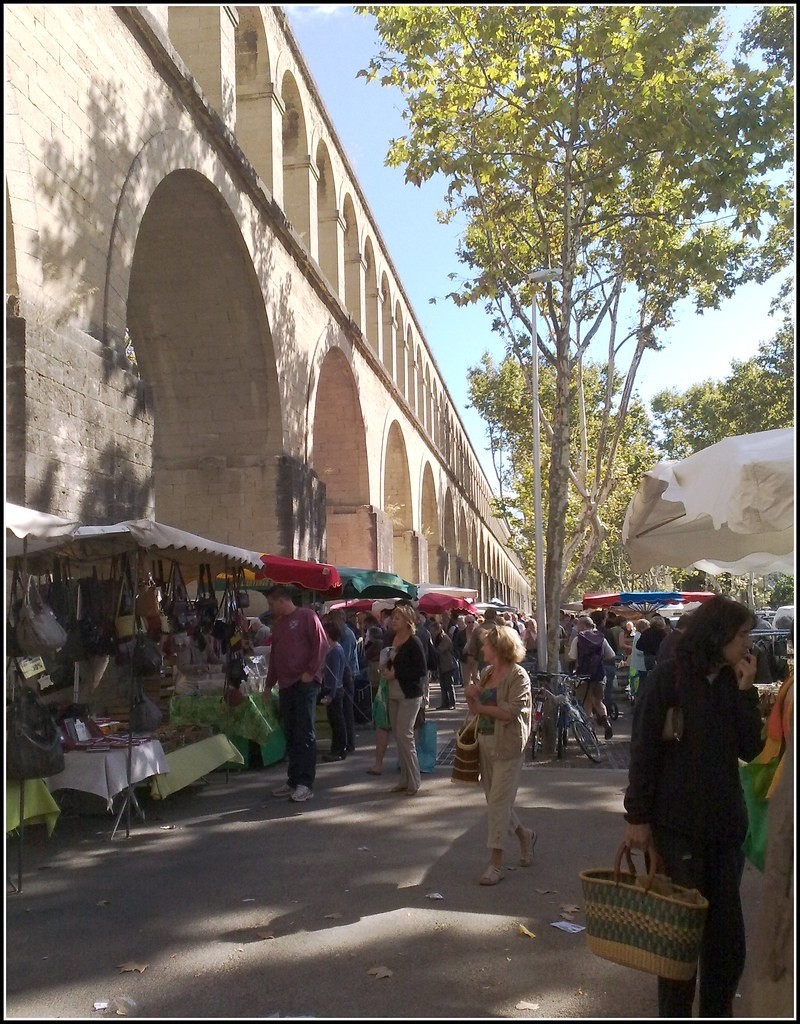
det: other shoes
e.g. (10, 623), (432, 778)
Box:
(365, 769), (382, 775)
(391, 786), (406, 792)
(603, 721), (613, 740)
(406, 789), (417, 796)
(346, 746), (355, 753)
(334, 750), (346, 761)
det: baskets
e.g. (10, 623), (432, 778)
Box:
(615, 660), (630, 687)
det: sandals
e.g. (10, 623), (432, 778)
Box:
(479, 865), (505, 886)
(520, 829), (537, 867)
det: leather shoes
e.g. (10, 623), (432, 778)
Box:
(448, 705), (455, 709)
(436, 705), (448, 710)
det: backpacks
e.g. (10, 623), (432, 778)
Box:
(577, 632), (605, 681)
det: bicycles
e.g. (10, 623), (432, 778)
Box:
(526, 647), (627, 764)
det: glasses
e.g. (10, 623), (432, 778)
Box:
(467, 621), (475, 624)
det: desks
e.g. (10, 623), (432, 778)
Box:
(5, 686), (283, 893)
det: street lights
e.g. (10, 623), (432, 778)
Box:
(526, 268), (565, 671)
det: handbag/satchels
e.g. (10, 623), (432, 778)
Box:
(416, 724), (437, 773)
(373, 669), (392, 730)
(6, 555), (250, 781)
(580, 841), (710, 981)
(659, 657), (684, 745)
(451, 711), (480, 786)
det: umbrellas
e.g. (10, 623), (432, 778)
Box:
(582, 591), (717, 622)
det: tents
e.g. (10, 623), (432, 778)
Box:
(6, 499), (419, 602)
(414, 582), (518, 616)
(620, 427), (794, 579)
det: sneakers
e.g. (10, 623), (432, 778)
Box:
(289, 784), (314, 801)
(270, 784), (295, 796)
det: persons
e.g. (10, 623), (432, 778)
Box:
(738, 620), (793, 1018)
(247, 616), (273, 648)
(449, 624), (538, 887)
(622, 593), (765, 1017)
(320, 599), (691, 797)
(171, 618), (228, 698)
(261, 584), (330, 803)
